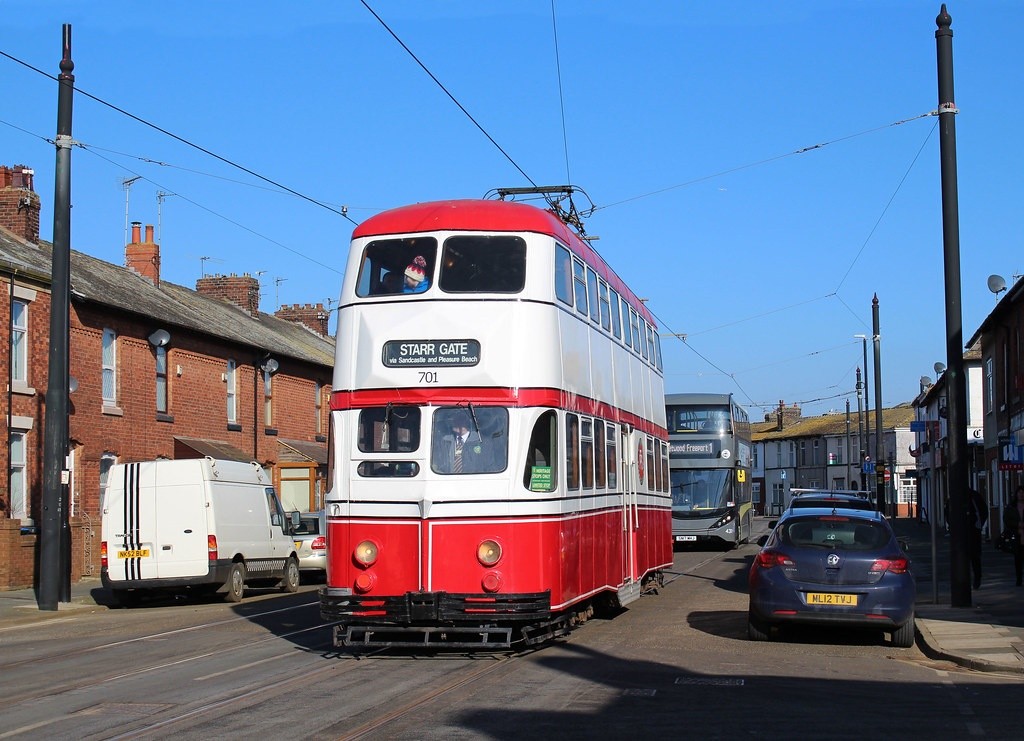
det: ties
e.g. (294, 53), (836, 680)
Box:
(454, 435), (462, 472)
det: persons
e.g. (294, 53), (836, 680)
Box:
(946, 480), (1024, 591)
(400, 257), (428, 294)
(435, 408), (497, 475)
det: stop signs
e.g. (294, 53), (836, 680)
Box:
(884, 469), (890, 482)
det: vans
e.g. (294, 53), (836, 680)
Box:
(99, 455), (302, 603)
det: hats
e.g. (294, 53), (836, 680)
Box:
(404, 255), (426, 281)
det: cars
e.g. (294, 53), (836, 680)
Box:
(788, 488), (875, 511)
(745, 505), (919, 647)
(270, 510), (327, 573)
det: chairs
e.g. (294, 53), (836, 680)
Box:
(790, 523), (881, 551)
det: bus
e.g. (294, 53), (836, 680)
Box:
(664, 392), (754, 550)
(318, 184), (675, 657)
